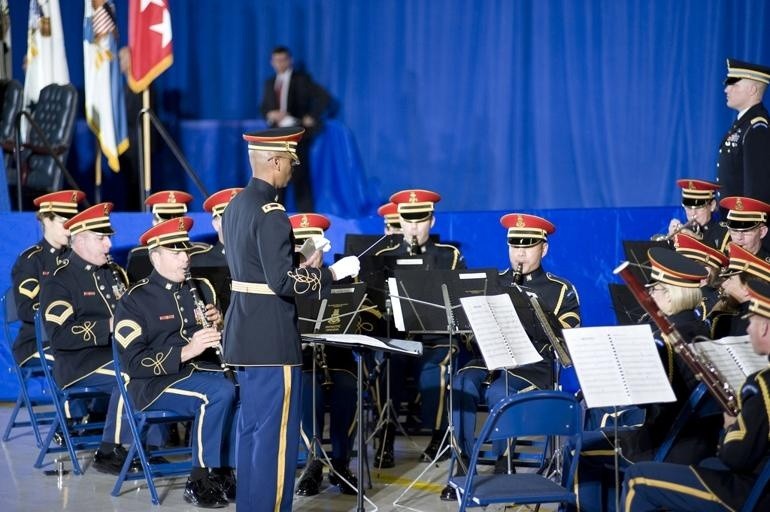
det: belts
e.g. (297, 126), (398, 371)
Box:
(230, 280), (276, 295)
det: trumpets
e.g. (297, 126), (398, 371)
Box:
(650, 215), (700, 242)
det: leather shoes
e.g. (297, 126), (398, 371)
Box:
(94, 446), (142, 476)
(78, 423), (180, 446)
(295, 460), (323, 497)
(328, 465), (366, 496)
(373, 440), (395, 468)
(53, 425), (84, 450)
(440, 482), (462, 502)
(211, 468), (236, 502)
(421, 440), (450, 463)
(135, 445), (172, 473)
(184, 478), (229, 508)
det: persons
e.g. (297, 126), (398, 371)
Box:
(259, 44), (342, 213)
(11, 186), (467, 508)
(222, 126), (361, 512)
(576, 179), (770, 512)
(440, 213), (582, 501)
(714, 58), (770, 204)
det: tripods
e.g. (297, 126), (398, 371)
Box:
(294, 344), (379, 512)
(392, 324), (468, 505)
(365, 308), (440, 491)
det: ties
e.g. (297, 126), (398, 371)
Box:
(275, 82), (282, 113)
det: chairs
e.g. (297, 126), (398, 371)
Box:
(296, 379), (374, 470)
(34, 308), (117, 476)
(448, 401), (555, 478)
(556, 367), (593, 399)
(650, 383), (723, 465)
(0, 83), (95, 215)
(448, 390), (583, 511)
(743, 459), (770, 511)
(2, 288), (80, 452)
(0, 82), (24, 172)
(111, 334), (197, 503)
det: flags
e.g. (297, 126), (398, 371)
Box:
(82, 0), (130, 174)
(19, 0), (71, 145)
(0, 0), (13, 82)
(123, 0), (174, 96)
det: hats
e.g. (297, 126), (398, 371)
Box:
(500, 214), (555, 248)
(644, 247), (709, 288)
(32, 190), (85, 219)
(202, 188), (244, 218)
(723, 57), (769, 87)
(63, 201), (116, 235)
(139, 217), (195, 252)
(145, 191), (193, 219)
(389, 190), (441, 224)
(673, 180), (770, 320)
(242, 127), (305, 167)
(289, 203), (401, 248)
